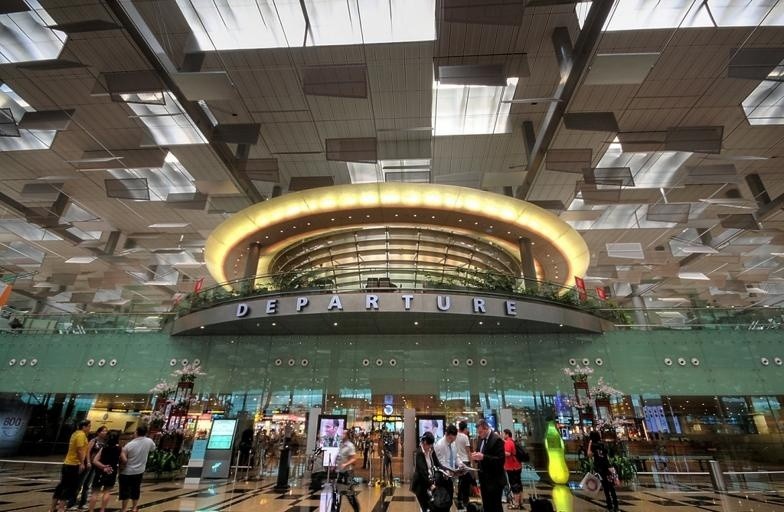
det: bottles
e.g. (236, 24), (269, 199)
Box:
(544, 418), (570, 484)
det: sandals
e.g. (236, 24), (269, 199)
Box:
(508, 504), (521, 509)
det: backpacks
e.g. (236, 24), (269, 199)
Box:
(511, 439), (529, 462)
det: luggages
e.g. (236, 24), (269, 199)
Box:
(320, 484), (333, 512)
(526, 465), (553, 512)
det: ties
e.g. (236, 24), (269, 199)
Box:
(449, 444), (453, 468)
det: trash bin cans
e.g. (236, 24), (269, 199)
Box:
(708, 459), (728, 494)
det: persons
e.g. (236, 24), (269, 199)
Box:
(361, 434), (373, 468)
(48, 419), (157, 512)
(419, 419), (442, 445)
(409, 418), (527, 511)
(592, 440), (620, 511)
(586, 426), (599, 458)
(320, 418), (339, 448)
(380, 426), (394, 485)
(238, 426), (299, 472)
(329, 430), (361, 511)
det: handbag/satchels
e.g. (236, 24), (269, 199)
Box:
(431, 488), (452, 510)
(609, 467), (620, 486)
(579, 472), (603, 497)
